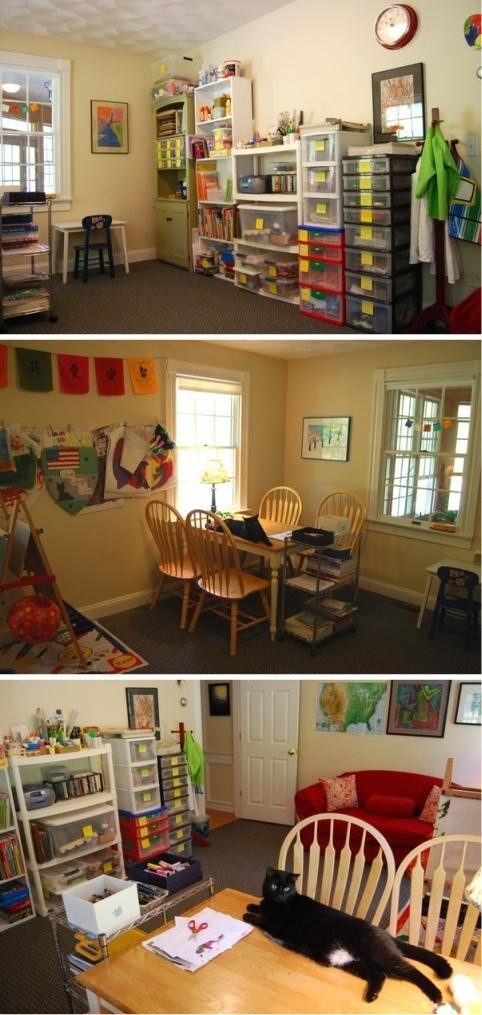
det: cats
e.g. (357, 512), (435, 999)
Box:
(243, 866), (454, 1005)
(215, 515), (274, 546)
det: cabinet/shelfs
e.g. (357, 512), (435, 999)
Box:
(151, 94), (198, 272)
(342, 153), (423, 334)
(0, 729), (192, 934)
(297, 131), (372, 327)
(233, 142), (298, 305)
(193, 76), (253, 284)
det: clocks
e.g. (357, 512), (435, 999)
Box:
(374, 4), (418, 50)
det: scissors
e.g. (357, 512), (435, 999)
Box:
(277, 126), (286, 137)
(186, 919), (208, 942)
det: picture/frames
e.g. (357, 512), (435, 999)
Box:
(371, 62), (425, 144)
(126, 687), (160, 741)
(91, 100), (129, 155)
(208, 683), (230, 717)
(300, 417), (351, 462)
(386, 680), (452, 738)
(454, 682), (481, 725)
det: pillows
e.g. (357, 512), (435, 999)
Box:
(417, 785), (441, 823)
(319, 774), (360, 812)
(366, 794), (416, 819)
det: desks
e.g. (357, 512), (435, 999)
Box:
(77, 887), (481, 1015)
(417, 558), (481, 629)
(52, 218), (129, 284)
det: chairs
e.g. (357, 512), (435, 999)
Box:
(278, 812), (396, 924)
(145, 485), (366, 657)
(427, 566), (481, 652)
(73, 215), (116, 283)
(389, 832), (482, 976)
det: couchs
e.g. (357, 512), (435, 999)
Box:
(294, 770), (462, 873)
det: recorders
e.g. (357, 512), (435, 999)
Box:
(12, 785), (56, 812)
(238, 174), (273, 194)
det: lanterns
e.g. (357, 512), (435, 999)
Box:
(6, 592), (62, 646)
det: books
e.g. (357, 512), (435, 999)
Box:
(285, 598), (358, 642)
(287, 550), (355, 596)
(271, 174), (297, 193)
(197, 170), (218, 201)
(0, 796), (32, 924)
(0, 222), (40, 252)
(31, 821), (52, 865)
(71, 948), (102, 967)
(197, 207), (242, 242)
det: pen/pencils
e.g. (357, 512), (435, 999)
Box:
(278, 110), (297, 132)
(137, 882), (164, 907)
(145, 861), (192, 876)
